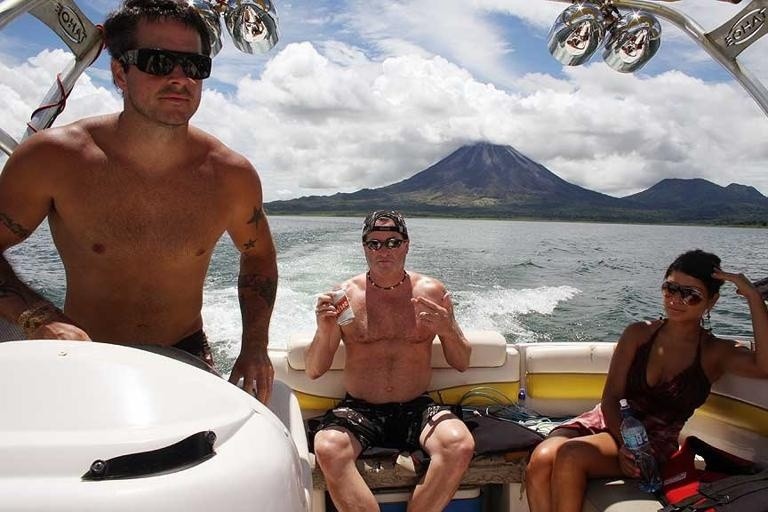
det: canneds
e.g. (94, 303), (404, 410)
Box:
(329, 288), (355, 327)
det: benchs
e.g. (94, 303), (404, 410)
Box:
(309, 450), (669, 512)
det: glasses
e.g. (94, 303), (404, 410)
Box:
(661, 281), (705, 306)
(362, 238), (408, 250)
(119, 48), (212, 80)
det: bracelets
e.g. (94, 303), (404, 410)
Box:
(16, 298), (63, 332)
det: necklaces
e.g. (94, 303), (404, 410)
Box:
(366, 268), (409, 291)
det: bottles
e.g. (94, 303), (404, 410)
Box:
(618, 398), (664, 493)
(518, 388), (527, 415)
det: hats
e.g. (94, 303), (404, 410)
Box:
(362, 210), (407, 237)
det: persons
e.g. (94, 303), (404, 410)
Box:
(523, 247), (767, 511)
(303, 208), (476, 511)
(1, 0), (280, 407)
